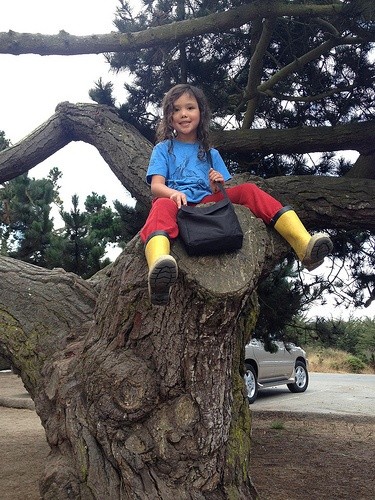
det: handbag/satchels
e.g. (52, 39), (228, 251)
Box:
(176, 198), (243, 257)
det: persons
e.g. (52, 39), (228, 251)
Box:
(140, 84), (333, 305)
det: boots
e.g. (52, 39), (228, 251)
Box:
(144, 231), (178, 305)
(270, 206), (333, 271)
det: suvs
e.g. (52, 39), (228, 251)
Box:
(244, 338), (308, 404)
